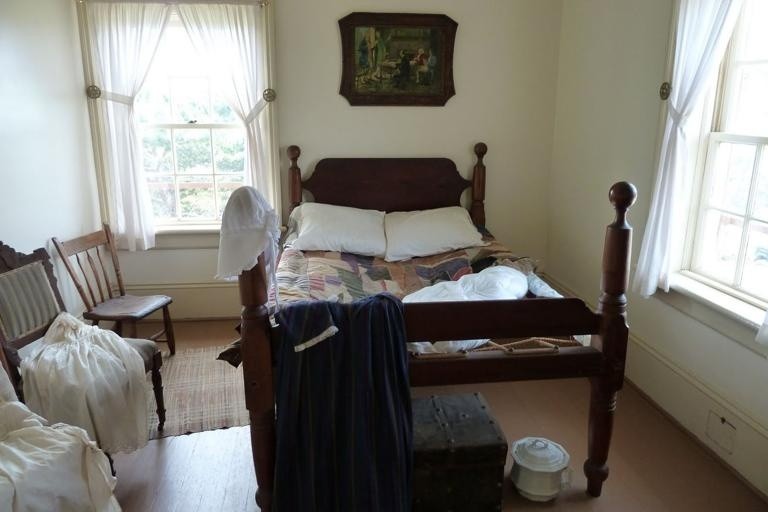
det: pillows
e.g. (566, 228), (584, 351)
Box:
(384, 206), (491, 262)
(287, 200), (386, 261)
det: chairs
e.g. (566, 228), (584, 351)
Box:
(1, 363), (123, 512)
(52, 224), (177, 359)
(1, 241), (166, 482)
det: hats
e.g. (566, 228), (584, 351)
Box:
(215, 186), (281, 280)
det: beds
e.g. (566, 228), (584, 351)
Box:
(231, 143), (639, 508)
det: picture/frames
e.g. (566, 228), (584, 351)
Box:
(338, 10), (459, 108)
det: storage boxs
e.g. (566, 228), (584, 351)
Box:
(410, 391), (509, 507)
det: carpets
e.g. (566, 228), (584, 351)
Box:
(107, 314), (253, 440)
